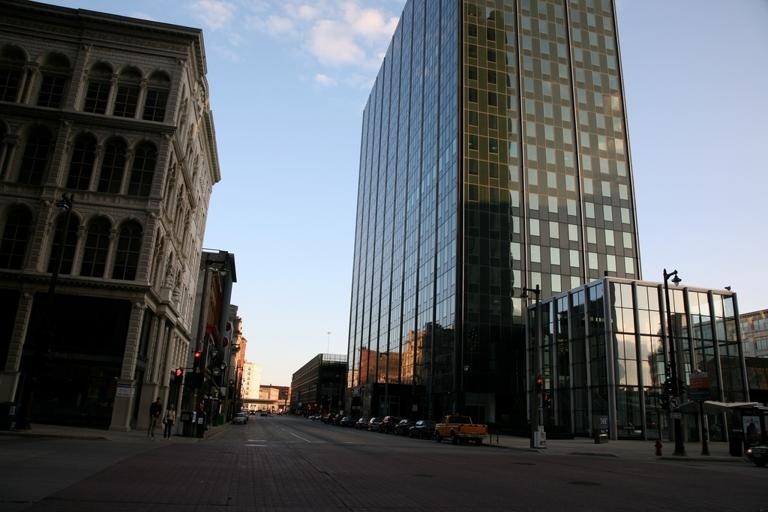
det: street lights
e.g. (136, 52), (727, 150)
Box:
(520, 283), (549, 449)
(203, 346), (220, 431)
(462, 364), (472, 417)
(379, 350), (389, 414)
(663, 265), (690, 456)
(11, 191), (77, 431)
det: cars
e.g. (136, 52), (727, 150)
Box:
(304, 412), (490, 444)
(744, 438), (768, 466)
(233, 409), (288, 425)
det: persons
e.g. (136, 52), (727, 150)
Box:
(163, 401), (176, 439)
(746, 417), (757, 434)
(147, 396), (162, 440)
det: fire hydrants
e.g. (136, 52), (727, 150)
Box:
(653, 439), (664, 457)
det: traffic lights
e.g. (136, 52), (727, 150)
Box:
(193, 349), (202, 370)
(536, 376), (542, 393)
(677, 377), (687, 394)
(666, 361), (671, 379)
(543, 392), (550, 402)
(174, 368), (183, 388)
